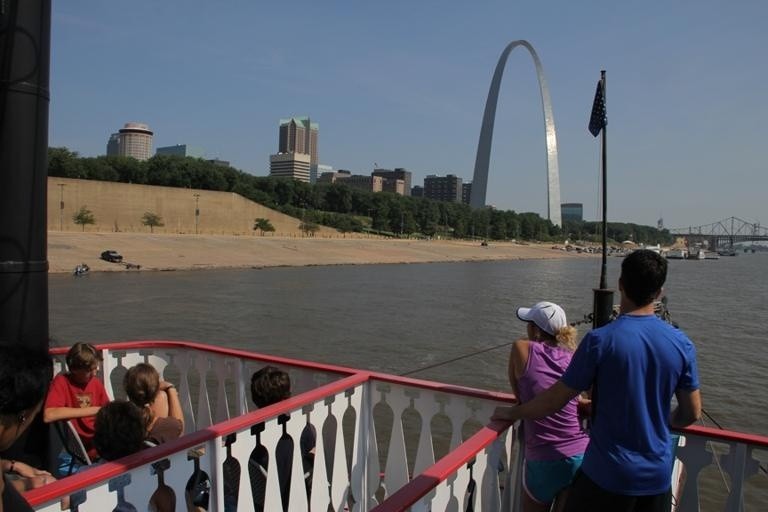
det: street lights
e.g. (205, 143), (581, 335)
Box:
(194, 193), (201, 235)
(57, 182), (67, 232)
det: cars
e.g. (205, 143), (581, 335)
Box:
(101, 249), (123, 263)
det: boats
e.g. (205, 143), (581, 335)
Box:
(74, 271), (89, 276)
(659, 248), (737, 259)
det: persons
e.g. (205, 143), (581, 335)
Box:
(490, 249), (701, 512)
(0, 343), (314, 512)
(509, 301), (591, 511)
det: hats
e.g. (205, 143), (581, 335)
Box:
(516, 302), (566, 336)
